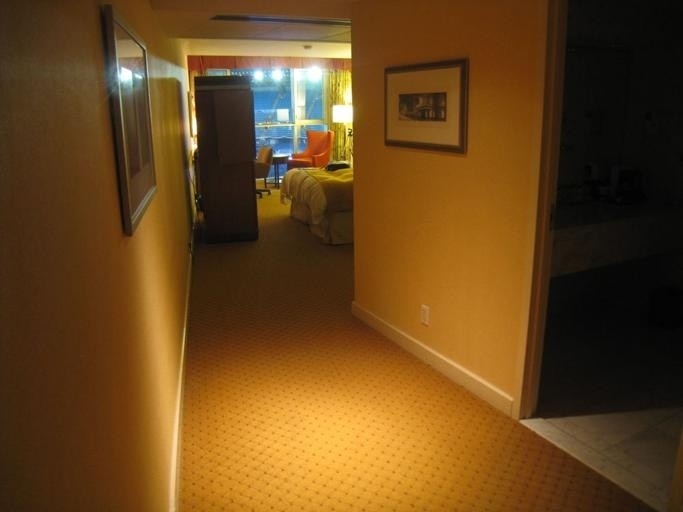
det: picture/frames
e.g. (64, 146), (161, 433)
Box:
(100, 2), (159, 237)
(383, 56), (468, 156)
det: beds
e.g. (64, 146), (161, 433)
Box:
(284, 163), (354, 247)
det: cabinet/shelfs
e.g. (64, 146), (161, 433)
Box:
(193, 74), (260, 243)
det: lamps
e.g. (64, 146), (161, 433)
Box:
(332, 103), (353, 160)
(276, 108), (289, 125)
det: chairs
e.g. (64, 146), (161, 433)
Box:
(252, 129), (335, 200)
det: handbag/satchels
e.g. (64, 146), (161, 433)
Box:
(325, 161), (349, 170)
